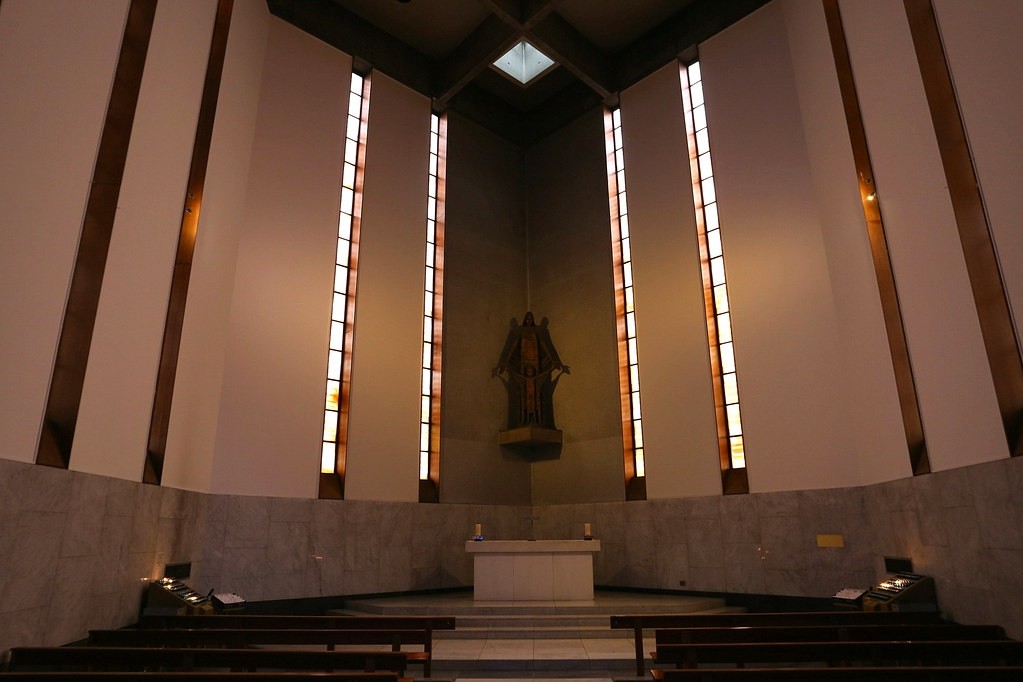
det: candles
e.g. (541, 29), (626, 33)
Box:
(475, 523), (481, 536)
(585, 522), (590, 536)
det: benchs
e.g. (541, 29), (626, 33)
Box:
(0, 610), (456, 682)
(610, 613), (1023, 682)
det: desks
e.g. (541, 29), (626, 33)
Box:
(465, 540), (600, 601)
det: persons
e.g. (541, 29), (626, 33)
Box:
(496, 311), (570, 426)
(500, 363), (555, 423)
